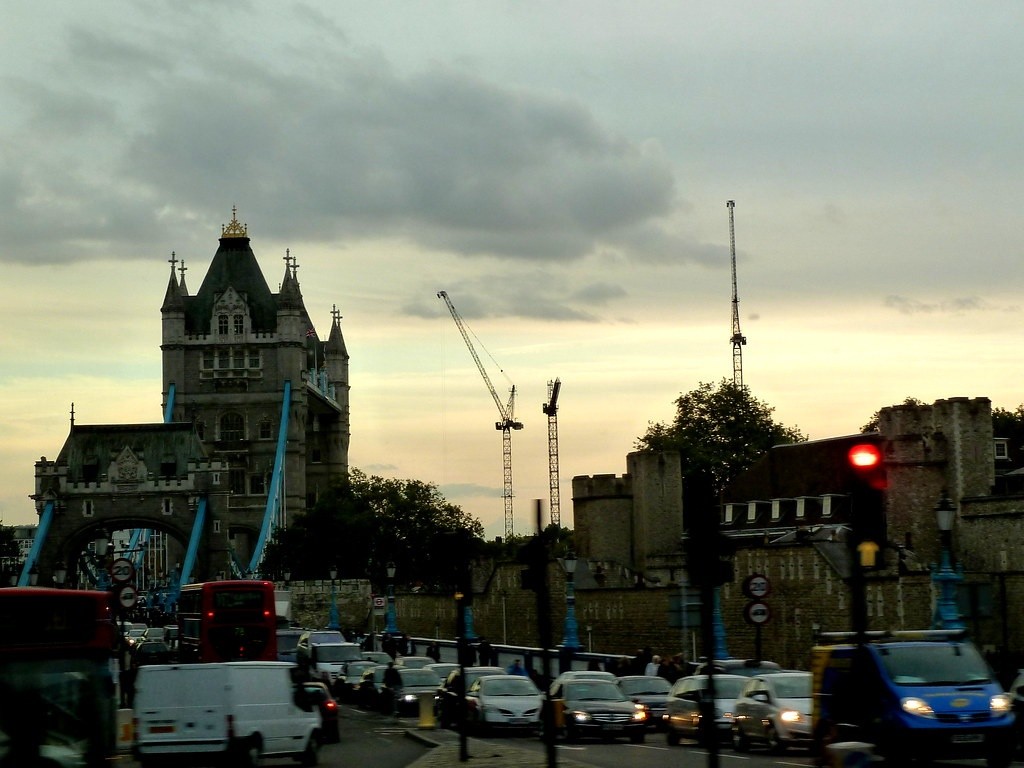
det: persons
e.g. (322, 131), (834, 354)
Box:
(381, 633), (398, 663)
(426, 642), (441, 663)
(397, 631), (416, 661)
(586, 648), (697, 684)
(508, 659), (529, 676)
(384, 662), (403, 713)
(364, 633), (377, 652)
(457, 636), (498, 668)
(338, 623), (365, 643)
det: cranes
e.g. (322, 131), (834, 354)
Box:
(727, 198), (747, 393)
(540, 376), (566, 533)
(435, 289), (522, 542)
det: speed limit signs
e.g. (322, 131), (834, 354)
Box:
(747, 573), (769, 598)
(112, 556), (137, 581)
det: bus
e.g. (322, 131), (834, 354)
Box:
(177, 579), (280, 665)
(0, 586), (124, 768)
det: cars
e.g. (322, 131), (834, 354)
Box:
(614, 673), (674, 731)
(464, 674), (547, 730)
(660, 672), (756, 745)
(545, 678), (649, 745)
(434, 665), (507, 730)
(548, 671), (617, 695)
(729, 667), (818, 753)
(116, 621), (184, 667)
(277, 628), (461, 717)
(293, 680), (340, 743)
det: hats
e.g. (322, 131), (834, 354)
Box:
(651, 655), (662, 661)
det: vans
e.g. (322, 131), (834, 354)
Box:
(691, 658), (782, 678)
(807, 626), (1020, 768)
(130, 658), (329, 768)
